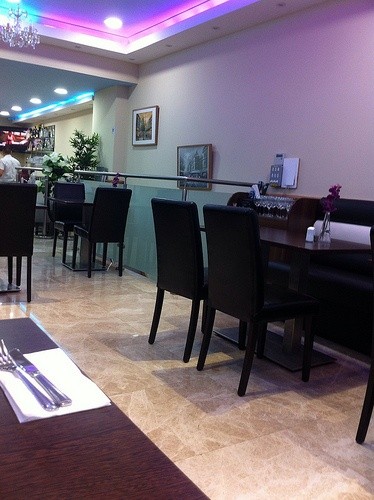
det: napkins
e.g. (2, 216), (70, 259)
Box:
(0, 348), (111, 423)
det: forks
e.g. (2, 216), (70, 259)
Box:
(0, 339), (58, 412)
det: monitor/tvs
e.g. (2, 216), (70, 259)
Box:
(0, 126), (32, 153)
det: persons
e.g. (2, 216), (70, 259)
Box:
(0, 145), (23, 183)
(6, 131), (16, 145)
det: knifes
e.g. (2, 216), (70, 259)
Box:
(10, 348), (72, 406)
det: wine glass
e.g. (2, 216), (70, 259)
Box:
(254, 195), (294, 221)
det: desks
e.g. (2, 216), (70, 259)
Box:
(47, 196), (108, 272)
(0, 318), (209, 500)
(202, 224), (374, 373)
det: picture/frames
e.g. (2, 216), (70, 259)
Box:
(176, 144), (212, 191)
(131, 105), (159, 147)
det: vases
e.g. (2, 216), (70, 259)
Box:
(317, 212), (331, 243)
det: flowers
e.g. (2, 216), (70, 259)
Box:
(319, 184), (342, 213)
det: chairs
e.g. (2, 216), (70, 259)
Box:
(53, 182), (85, 262)
(197, 204), (324, 397)
(72, 187), (131, 278)
(147, 197), (246, 363)
(0, 180), (38, 302)
